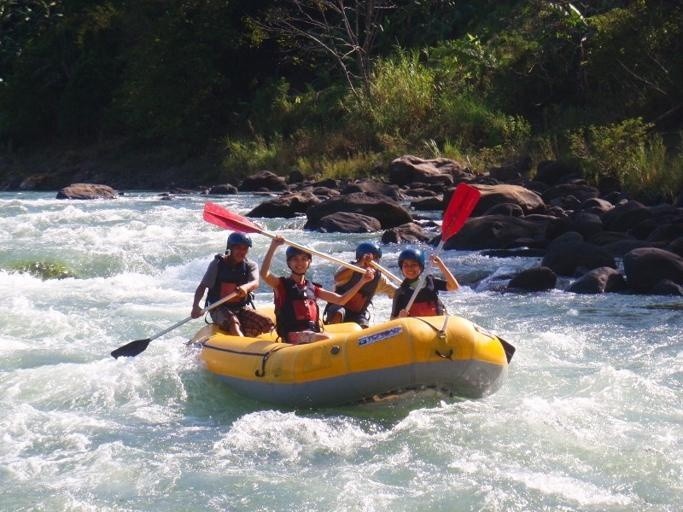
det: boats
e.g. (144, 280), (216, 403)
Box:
(182, 303), (509, 411)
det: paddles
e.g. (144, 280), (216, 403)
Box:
(203, 203), (373, 275)
(111, 290), (237, 360)
(405, 182), (481, 311)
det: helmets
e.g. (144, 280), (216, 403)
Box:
(398, 248), (425, 269)
(285, 243), (312, 263)
(227, 231), (252, 249)
(356, 242), (382, 259)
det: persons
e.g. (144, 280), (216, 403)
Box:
(190, 230), (274, 338)
(389, 247), (460, 321)
(259, 233), (374, 346)
(323, 242), (401, 330)
